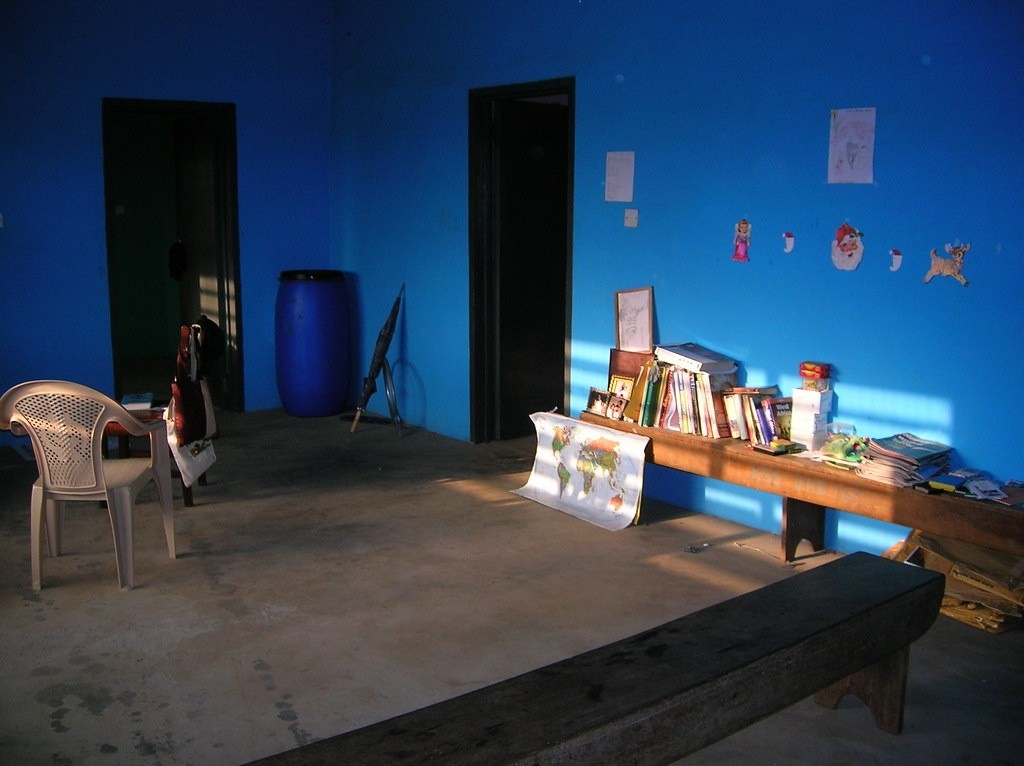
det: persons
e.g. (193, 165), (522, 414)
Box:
(592, 394), (623, 420)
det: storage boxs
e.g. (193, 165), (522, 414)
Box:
(881, 528), (1024, 636)
(789, 388), (833, 451)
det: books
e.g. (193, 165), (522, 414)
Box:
(623, 342), (1024, 506)
(121, 392), (154, 411)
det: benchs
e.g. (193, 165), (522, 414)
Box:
(580, 413), (1023, 562)
(240, 552), (947, 766)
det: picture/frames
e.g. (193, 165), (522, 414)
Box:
(614, 286), (654, 355)
(586, 374), (636, 421)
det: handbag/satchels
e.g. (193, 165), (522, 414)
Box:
(171, 325), (222, 447)
(165, 396), (217, 488)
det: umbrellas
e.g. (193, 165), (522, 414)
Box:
(341, 415), (391, 424)
(381, 356), (403, 438)
(347, 291), (403, 435)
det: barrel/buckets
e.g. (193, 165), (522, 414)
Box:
(274, 270), (352, 418)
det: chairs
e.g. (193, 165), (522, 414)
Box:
(65, 315), (207, 508)
(0, 380), (178, 591)
